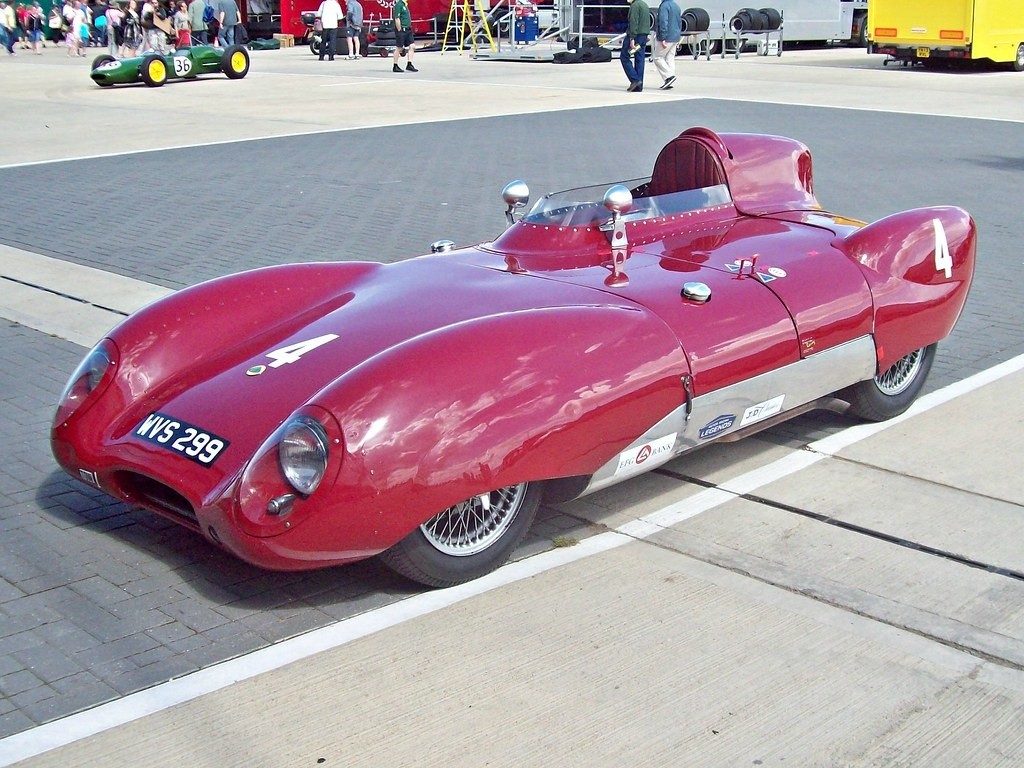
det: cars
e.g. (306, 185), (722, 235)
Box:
(88, 39), (254, 87)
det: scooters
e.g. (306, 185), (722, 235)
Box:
(300, 10), (347, 53)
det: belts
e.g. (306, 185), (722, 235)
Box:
(402, 27), (411, 30)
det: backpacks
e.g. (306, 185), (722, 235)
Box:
(203, 0), (214, 24)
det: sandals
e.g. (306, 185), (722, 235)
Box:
(344, 55), (354, 60)
(353, 55), (362, 60)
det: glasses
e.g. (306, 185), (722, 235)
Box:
(170, 3), (174, 5)
(151, 2), (157, 5)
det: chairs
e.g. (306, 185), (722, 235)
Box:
(651, 137), (729, 211)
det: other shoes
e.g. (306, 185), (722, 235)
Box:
(8, 51), (17, 56)
(20, 43), (26, 49)
(24, 42), (31, 49)
(130, 53), (137, 57)
(329, 56), (334, 61)
(627, 80), (642, 92)
(319, 55), (325, 61)
(120, 54), (125, 57)
(65, 51), (87, 57)
(33, 51), (42, 55)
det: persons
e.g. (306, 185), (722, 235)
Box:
(620, 0), (652, 92)
(390, 0), (420, 72)
(651, 0), (682, 90)
(317, 0), (344, 61)
(342, 0), (364, 60)
(0, 0), (242, 58)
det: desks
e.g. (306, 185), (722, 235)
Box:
(649, 30), (710, 62)
(721, 10), (784, 59)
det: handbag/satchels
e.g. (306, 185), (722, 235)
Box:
(237, 23), (249, 44)
(61, 24), (70, 31)
(115, 26), (124, 46)
(49, 17), (61, 28)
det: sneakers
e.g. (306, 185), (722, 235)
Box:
(406, 65), (418, 72)
(393, 65), (404, 72)
(660, 76), (677, 89)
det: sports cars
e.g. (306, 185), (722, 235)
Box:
(49, 127), (974, 590)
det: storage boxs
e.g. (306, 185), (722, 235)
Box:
(757, 40), (778, 56)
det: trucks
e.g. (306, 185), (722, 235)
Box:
(868, 0), (1024, 72)
(556, 0), (867, 56)
(232, 0), (554, 43)
